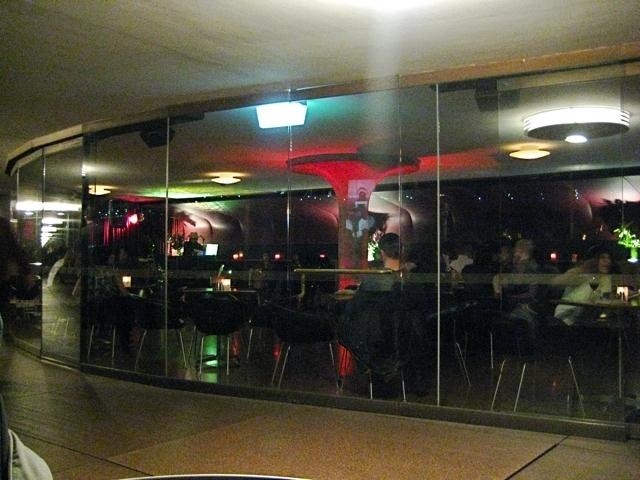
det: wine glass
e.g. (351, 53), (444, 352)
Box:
(589, 277), (601, 302)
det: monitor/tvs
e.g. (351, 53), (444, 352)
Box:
(205, 243), (219, 256)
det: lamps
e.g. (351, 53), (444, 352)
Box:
(521, 103), (630, 145)
(256, 101), (307, 130)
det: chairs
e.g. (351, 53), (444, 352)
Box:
(266, 299), (473, 401)
(86, 293), (259, 374)
(481, 294), (587, 415)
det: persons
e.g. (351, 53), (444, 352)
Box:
(183, 232), (204, 255)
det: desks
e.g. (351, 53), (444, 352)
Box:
(562, 296), (639, 401)
